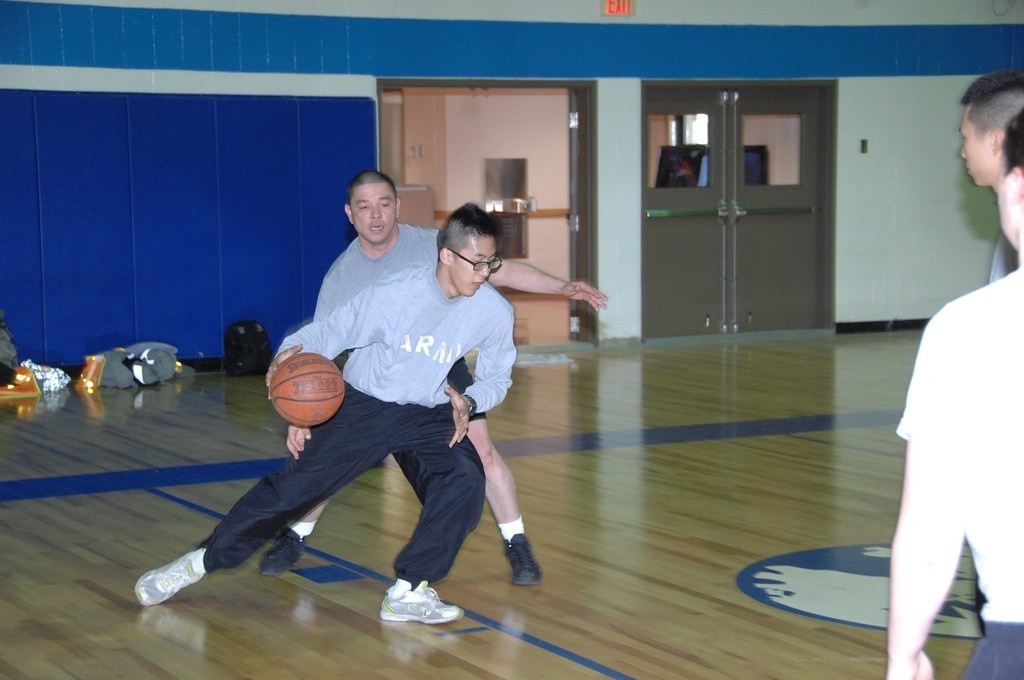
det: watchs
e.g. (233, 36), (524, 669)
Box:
(464, 395), (476, 415)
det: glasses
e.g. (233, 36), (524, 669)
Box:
(449, 248), (502, 272)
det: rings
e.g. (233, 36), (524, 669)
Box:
(467, 428), (468, 431)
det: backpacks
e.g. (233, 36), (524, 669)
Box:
(222, 319), (273, 377)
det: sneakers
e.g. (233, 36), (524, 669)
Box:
(258, 526), (307, 577)
(502, 532), (541, 586)
(380, 580), (464, 625)
(133, 551), (200, 606)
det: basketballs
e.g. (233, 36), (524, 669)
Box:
(270, 352), (346, 428)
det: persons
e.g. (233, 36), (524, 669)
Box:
(887, 112), (1024, 680)
(259, 169), (608, 586)
(959, 70), (1024, 638)
(134, 203), (518, 624)
